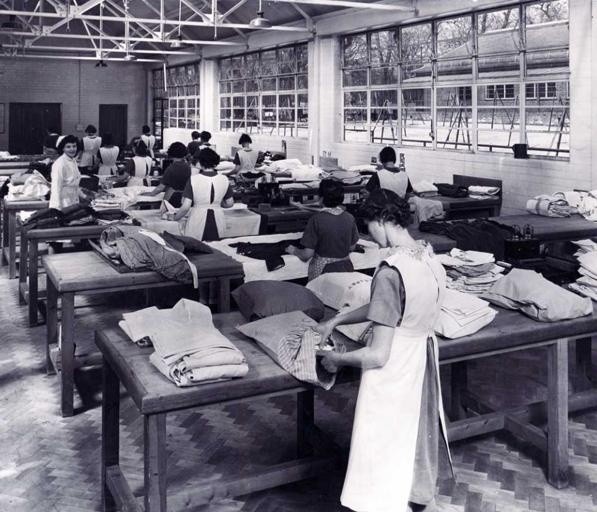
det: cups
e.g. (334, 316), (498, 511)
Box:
(521, 223), (535, 239)
(265, 155), (271, 161)
(99, 175), (114, 192)
(512, 223), (522, 241)
(327, 151), (330, 157)
(322, 151), (326, 157)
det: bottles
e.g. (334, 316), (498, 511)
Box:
(153, 170), (158, 177)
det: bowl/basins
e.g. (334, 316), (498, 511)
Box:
(155, 121), (162, 142)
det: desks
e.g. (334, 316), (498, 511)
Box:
(0, 149), (597, 511)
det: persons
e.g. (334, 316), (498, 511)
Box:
(282, 178), (361, 281)
(362, 146), (418, 201)
(310, 188), (455, 511)
(40, 122), (268, 244)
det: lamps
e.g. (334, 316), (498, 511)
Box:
(9, 0), (270, 70)
